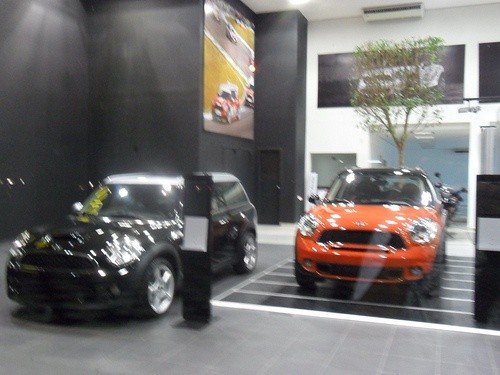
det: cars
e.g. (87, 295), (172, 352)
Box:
(295, 168), (447, 296)
(6, 172), (258, 318)
(207, 3), (254, 124)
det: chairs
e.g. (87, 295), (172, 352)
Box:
(355, 181), (381, 200)
(401, 183), (422, 203)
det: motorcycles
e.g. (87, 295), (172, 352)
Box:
(434, 171), (467, 219)
